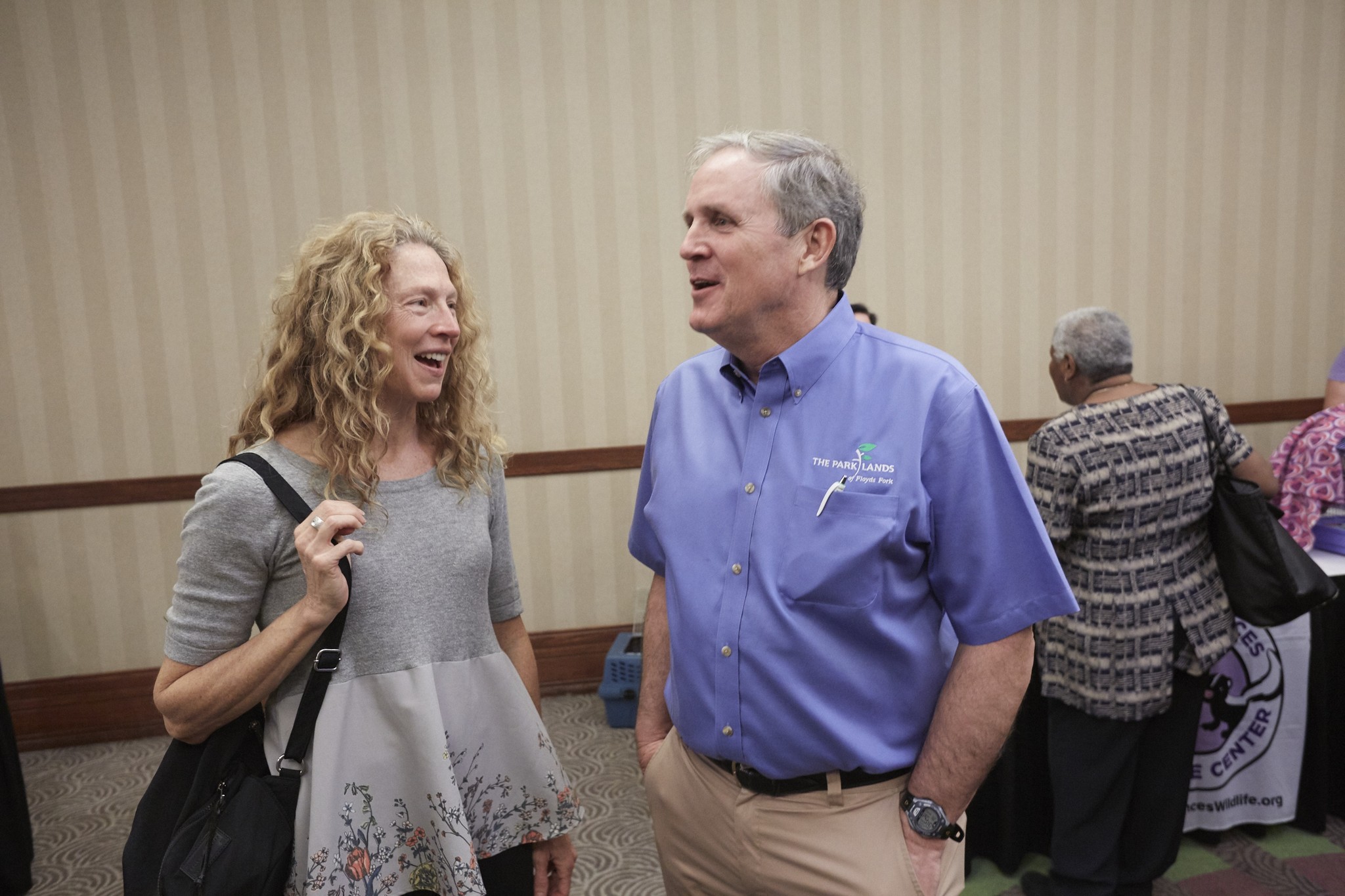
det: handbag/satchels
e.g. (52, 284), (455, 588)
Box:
(121, 699), (308, 896)
(1210, 474), (1340, 629)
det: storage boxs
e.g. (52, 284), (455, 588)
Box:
(1311, 514), (1345, 556)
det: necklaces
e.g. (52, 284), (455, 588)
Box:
(1088, 382), (1128, 400)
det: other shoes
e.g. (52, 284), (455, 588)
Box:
(976, 816), (1026, 875)
(1022, 870), (1059, 896)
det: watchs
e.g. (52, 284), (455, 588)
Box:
(900, 787), (964, 843)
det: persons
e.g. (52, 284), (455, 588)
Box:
(628, 130), (1078, 896)
(152, 214), (578, 896)
(1323, 344), (1345, 410)
(850, 304), (877, 326)
(1025, 307), (1282, 896)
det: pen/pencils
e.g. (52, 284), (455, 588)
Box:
(816, 475), (848, 516)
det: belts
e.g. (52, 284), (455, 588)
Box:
(705, 753), (915, 798)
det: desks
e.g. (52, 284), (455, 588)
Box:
(965, 548), (1345, 874)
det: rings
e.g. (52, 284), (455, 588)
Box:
(310, 517), (325, 530)
